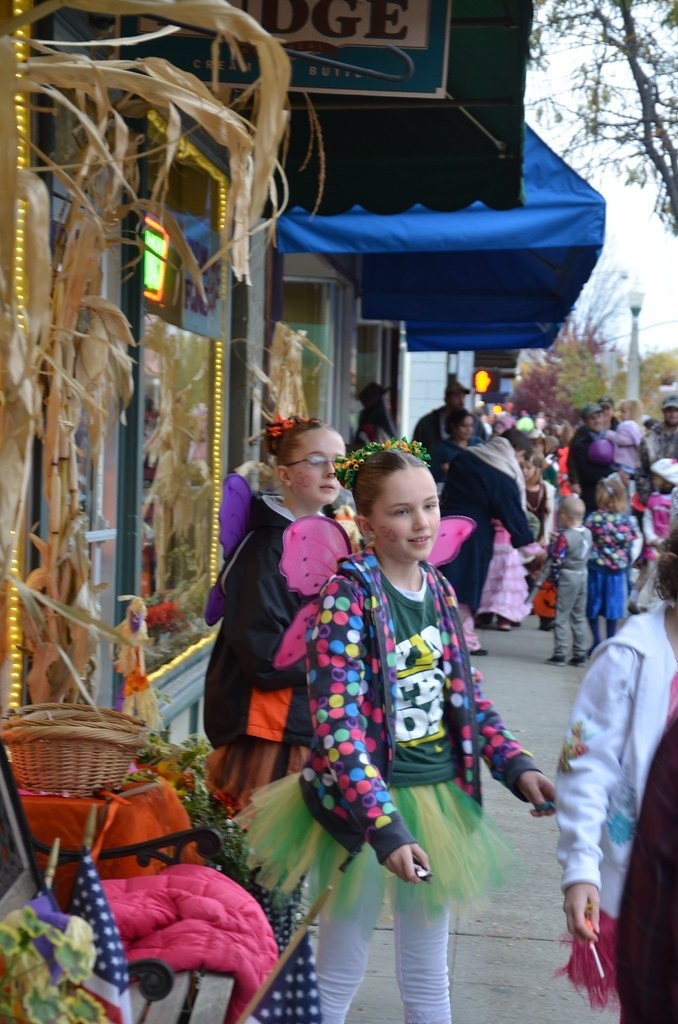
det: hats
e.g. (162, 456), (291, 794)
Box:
(598, 396), (614, 408)
(445, 382), (470, 396)
(661, 394), (678, 410)
(357, 381), (392, 406)
(587, 438), (614, 466)
(581, 403), (603, 417)
(444, 407), (474, 435)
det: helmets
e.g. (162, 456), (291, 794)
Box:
(651, 458), (678, 486)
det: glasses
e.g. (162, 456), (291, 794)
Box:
(284, 453), (339, 466)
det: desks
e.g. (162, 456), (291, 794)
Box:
(17, 763), (206, 915)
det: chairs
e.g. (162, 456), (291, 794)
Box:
(0, 733), (274, 1024)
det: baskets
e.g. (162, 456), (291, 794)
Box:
(0, 702), (147, 798)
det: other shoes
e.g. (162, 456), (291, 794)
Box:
(509, 620), (521, 627)
(470, 649), (488, 656)
(547, 657), (565, 665)
(567, 658), (586, 666)
(496, 621), (511, 630)
(539, 626), (550, 631)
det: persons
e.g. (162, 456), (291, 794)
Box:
(203, 376), (678, 1023)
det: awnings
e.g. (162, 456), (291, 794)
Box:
(270, 120), (606, 351)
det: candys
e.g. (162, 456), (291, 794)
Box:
(412, 858), (432, 880)
(584, 918), (605, 979)
(533, 800), (556, 811)
